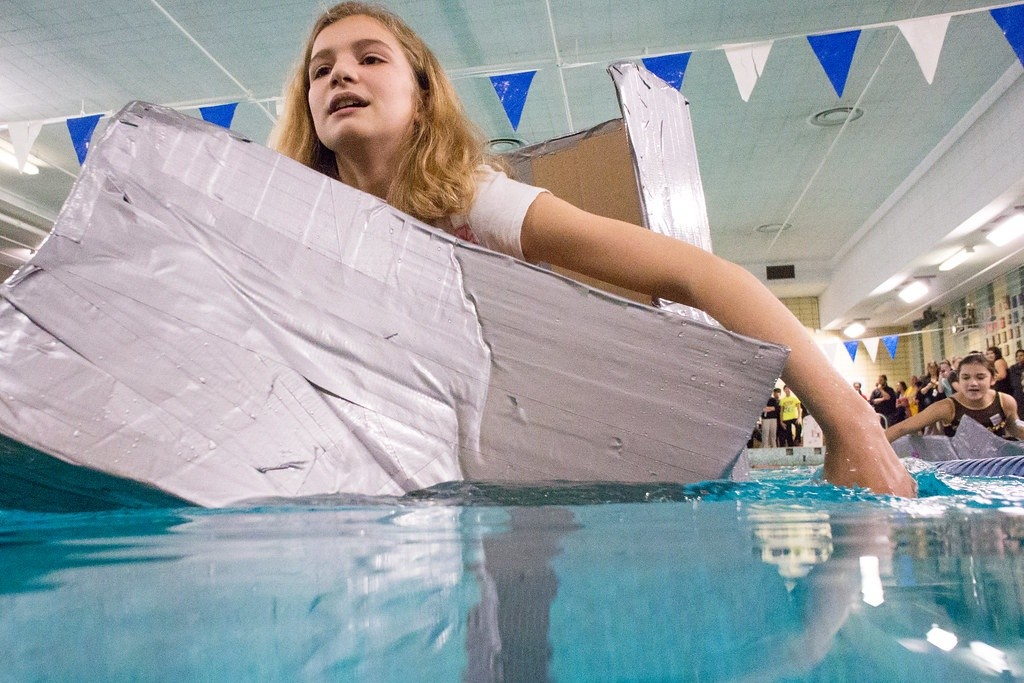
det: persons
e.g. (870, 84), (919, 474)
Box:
(761, 346), (1024, 450)
(269, 0), (919, 501)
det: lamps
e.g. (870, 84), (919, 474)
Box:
(939, 247), (975, 271)
